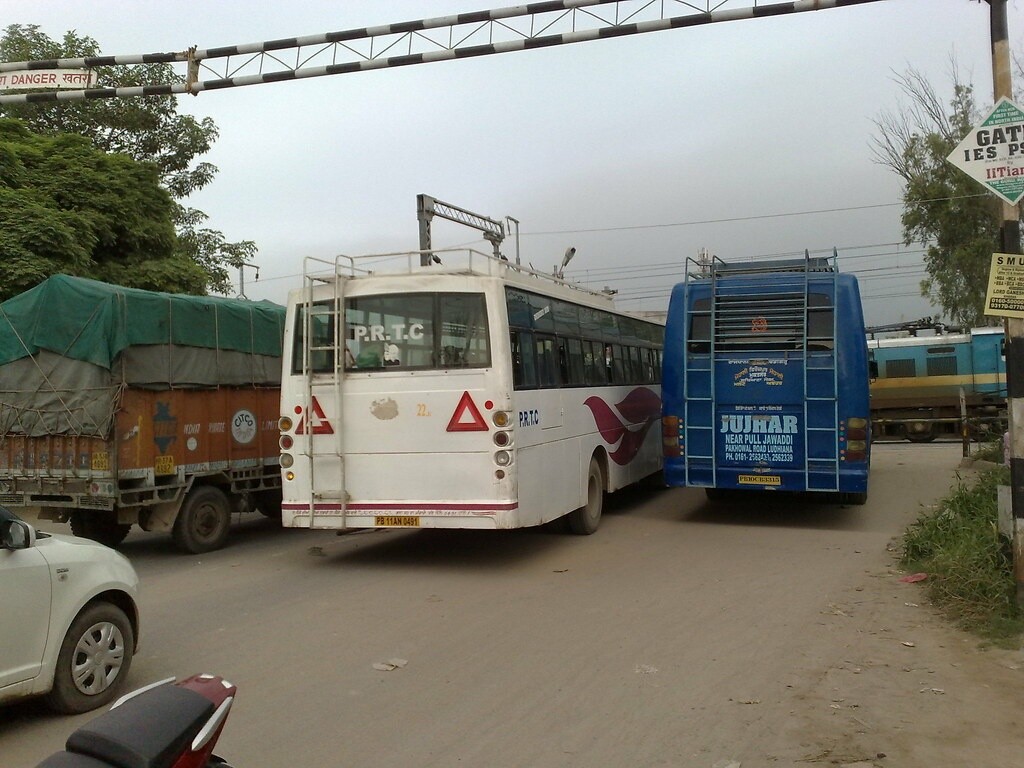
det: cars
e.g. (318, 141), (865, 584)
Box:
(0, 503), (142, 717)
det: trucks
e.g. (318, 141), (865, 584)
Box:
(0, 272), (357, 554)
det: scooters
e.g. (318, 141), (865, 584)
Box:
(34, 671), (237, 767)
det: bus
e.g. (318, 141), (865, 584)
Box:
(865, 325), (1007, 444)
(273, 244), (667, 536)
(658, 245), (879, 507)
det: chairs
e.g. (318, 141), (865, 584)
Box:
(432, 346), (468, 365)
(606, 358), (661, 383)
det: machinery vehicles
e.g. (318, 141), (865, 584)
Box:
(864, 315), (970, 335)
